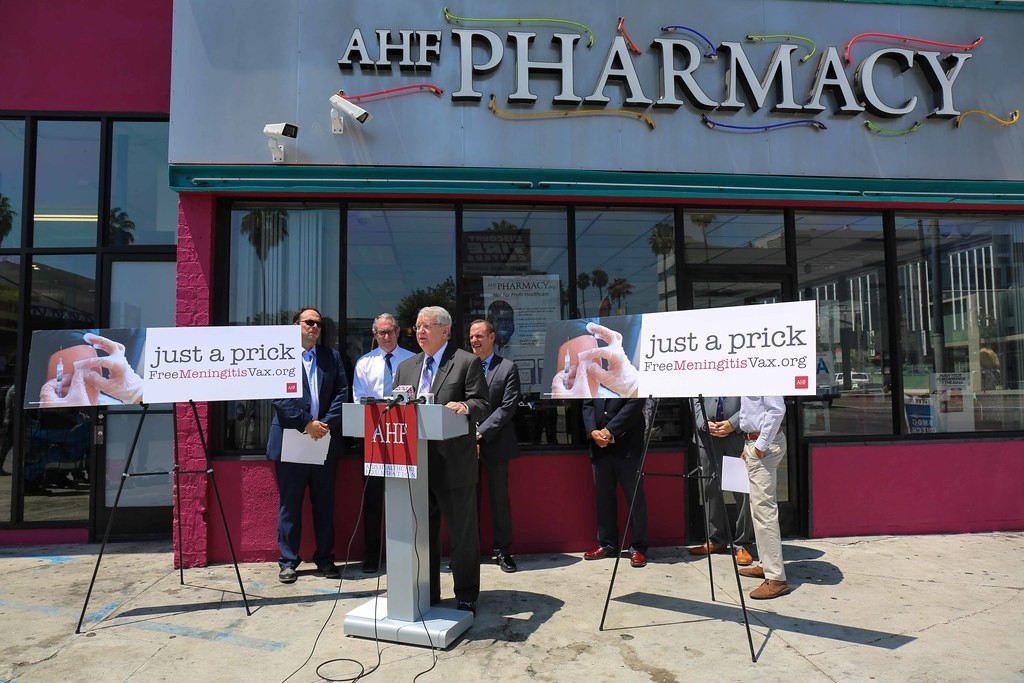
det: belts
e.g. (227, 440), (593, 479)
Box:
(742, 426), (783, 441)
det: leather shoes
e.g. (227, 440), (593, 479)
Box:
(736, 548), (753, 566)
(630, 552), (647, 567)
(583, 546), (608, 559)
(318, 563), (341, 578)
(738, 567), (766, 579)
(278, 567), (297, 582)
(688, 542), (727, 554)
(496, 556), (517, 572)
(749, 579), (791, 599)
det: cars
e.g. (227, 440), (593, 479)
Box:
(834, 373), (870, 389)
(851, 381), (911, 401)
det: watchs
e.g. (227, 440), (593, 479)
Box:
(476, 431), (482, 438)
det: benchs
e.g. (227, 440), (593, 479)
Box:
(34, 209), (98, 222)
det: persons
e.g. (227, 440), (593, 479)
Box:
(739, 396), (789, 598)
(392, 306), (491, 617)
(550, 322), (639, 399)
(486, 300), (515, 359)
(266, 307), (348, 582)
(352, 313), (417, 572)
(582, 398), (649, 566)
(688, 397), (754, 564)
(24, 330), (102, 408)
(532, 399), (558, 444)
(540, 319), (602, 399)
(979, 348), (1002, 421)
(449, 319), (520, 572)
(0, 384), (16, 476)
(39, 332), (144, 408)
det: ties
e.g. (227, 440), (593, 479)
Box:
(480, 361), (486, 377)
(418, 357), (434, 393)
(383, 353), (394, 397)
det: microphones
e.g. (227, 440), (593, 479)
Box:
(382, 384), (415, 414)
(406, 393), (434, 405)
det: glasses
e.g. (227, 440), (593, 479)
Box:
(376, 329), (397, 335)
(469, 334), (488, 339)
(413, 322), (443, 331)
(296, 320), (323, 328)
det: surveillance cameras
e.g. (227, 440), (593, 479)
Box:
(264, 123), (299, 139)
(329, 94), (370, 125)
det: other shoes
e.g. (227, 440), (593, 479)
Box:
(362, 561), (380, 572)
